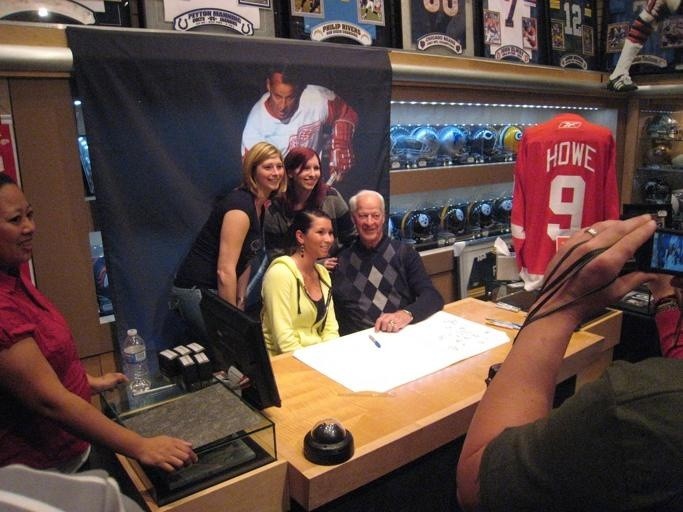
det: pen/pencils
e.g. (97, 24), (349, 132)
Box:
(485, 318), (521, 330)
(369, 335), (381, 348)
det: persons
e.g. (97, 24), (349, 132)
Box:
(508, 106), (618, 295)
(169, 140), (288, 370)
(0, 173), (200, 482)
(604, 0), (683, 93)
(235, 64), (358, 188)
(656, 233), (681, 271)
(259, 207), (342, 360)
(262, 146), (356, 270)
(328, 189), (445, 340)
(454, 212), (680, 510)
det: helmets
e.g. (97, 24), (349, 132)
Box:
(390, 198), (511, 242)
(388, 126), (522, 168)
(639, 115), (683, 221)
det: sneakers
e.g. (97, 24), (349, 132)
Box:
(608, 72), (639, 93)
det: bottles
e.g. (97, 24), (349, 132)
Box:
(122, 330), (148, 389)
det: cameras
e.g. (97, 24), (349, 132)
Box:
(636, 229), (683, 277)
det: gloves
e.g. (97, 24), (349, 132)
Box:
(329, 119), (357, 181)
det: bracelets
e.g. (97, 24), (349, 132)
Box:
(399, 308), (414, 320)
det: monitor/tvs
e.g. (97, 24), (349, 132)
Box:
(199, 285), (281, 411)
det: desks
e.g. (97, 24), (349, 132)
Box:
(250, 297), (605, 511)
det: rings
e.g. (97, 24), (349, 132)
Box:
(389, 320), (396, 326)
(586, 226), (597, 237)
